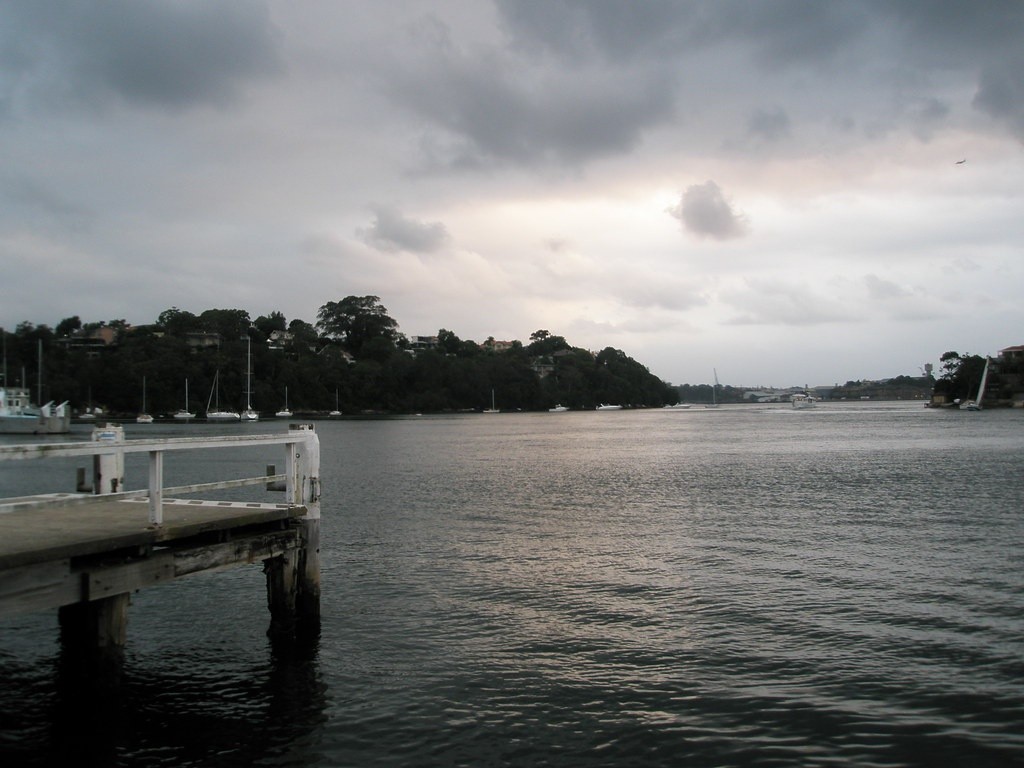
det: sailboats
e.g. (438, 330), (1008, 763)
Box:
(241, 336), (259, 420)
(174, 378), (196, 418)
(206, 370), (240, 420)
(483, 389), (500, 412)
(79, 385), (97, 418)
(136, 378), (154, 423)
(276, 385), (293, 417)
(329, 389), (343, 416)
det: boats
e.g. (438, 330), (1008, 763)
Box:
(0, 384), (70, 433)
(663, 402), (691, 409)
(792, 392), (817, 408)
(595, 403), (623, 410)
(549, 405), (570, 412)
(959, 401), (984, 411)
(704, 403), (720, 408)
